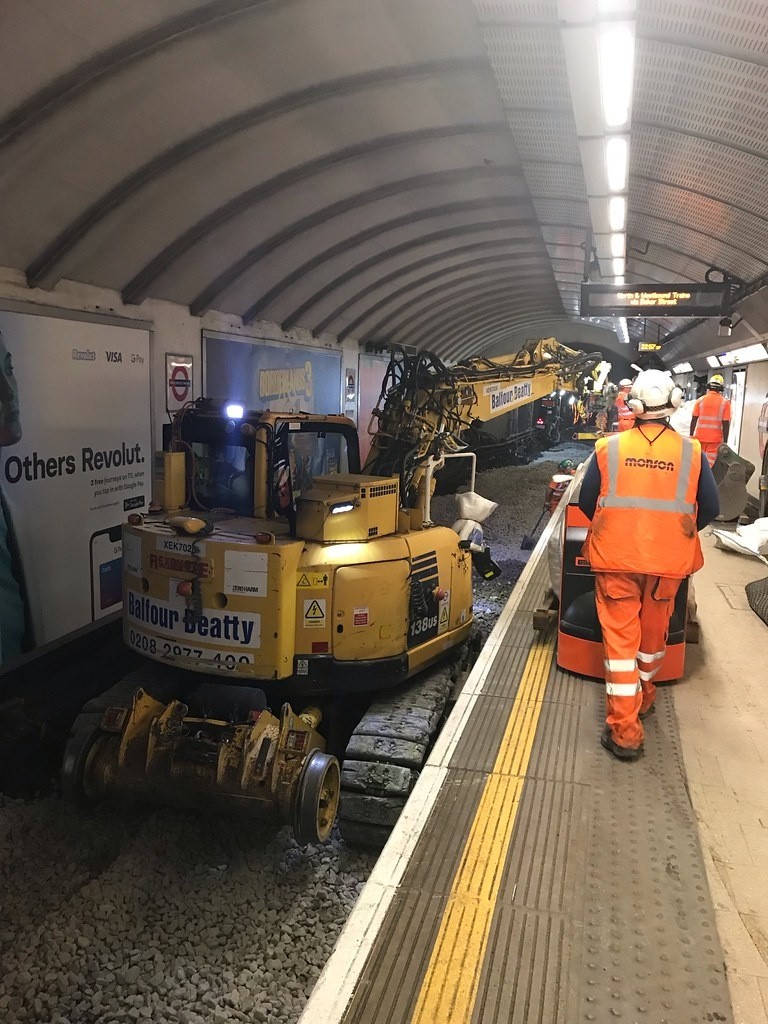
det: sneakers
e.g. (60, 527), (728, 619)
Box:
(638, 704), (655, 720)
(601, 726), (644, 763)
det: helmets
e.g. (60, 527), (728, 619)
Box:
(707, 373), (725, 392)
(625, 369), (684, 421)
(559, 459), (576, 472)
(619, 378), (633, 389)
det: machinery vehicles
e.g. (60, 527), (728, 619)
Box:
(50, 337), (604, 845)
(569, 380), (617, 441)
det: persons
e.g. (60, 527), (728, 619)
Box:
(690, 374), (732, 469)
(615, 379), (637, 430)
(543, 460), (576, 516)
(579, 369), (720, 759)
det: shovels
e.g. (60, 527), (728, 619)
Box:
(521, 503), (551, 550)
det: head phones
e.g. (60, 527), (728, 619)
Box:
(704, 382), (725, 392)
(623, 386), (687, 415)
